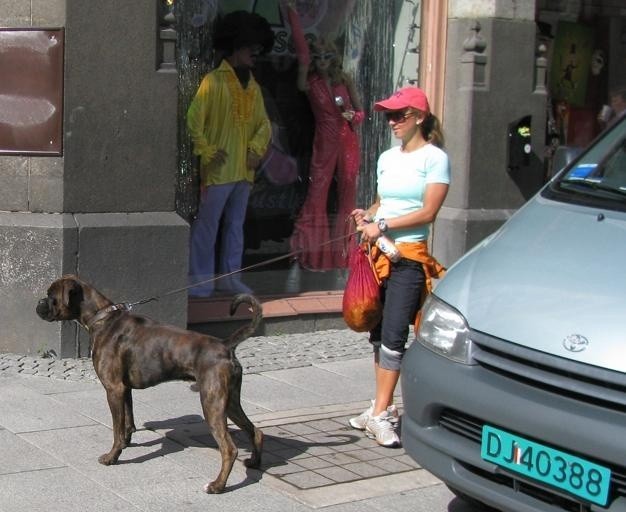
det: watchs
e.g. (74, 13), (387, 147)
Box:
(378, 216), (388, 233)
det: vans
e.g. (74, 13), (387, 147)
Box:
(389, 105), (626, 511)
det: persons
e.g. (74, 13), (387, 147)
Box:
(185, 11), (276, 300)
(349, 87), (450, 446)
(596, 87), (626, 130)
(290, 7), (366, 272)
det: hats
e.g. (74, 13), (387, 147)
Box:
(373, 87), (429, 112)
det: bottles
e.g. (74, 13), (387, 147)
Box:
(369, 231), (401, 264)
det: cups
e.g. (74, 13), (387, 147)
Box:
(600, 104), (614, 121)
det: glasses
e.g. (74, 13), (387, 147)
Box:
(313, 54), (332, 59)
(385, 112), (417, 123)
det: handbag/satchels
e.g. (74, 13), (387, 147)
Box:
(343, 246), (382, 333)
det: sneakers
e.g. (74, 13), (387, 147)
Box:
(366, 411), (401, 447)
(349, 398), (399, 430)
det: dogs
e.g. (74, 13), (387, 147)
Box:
(36, 274), (265, 496)
